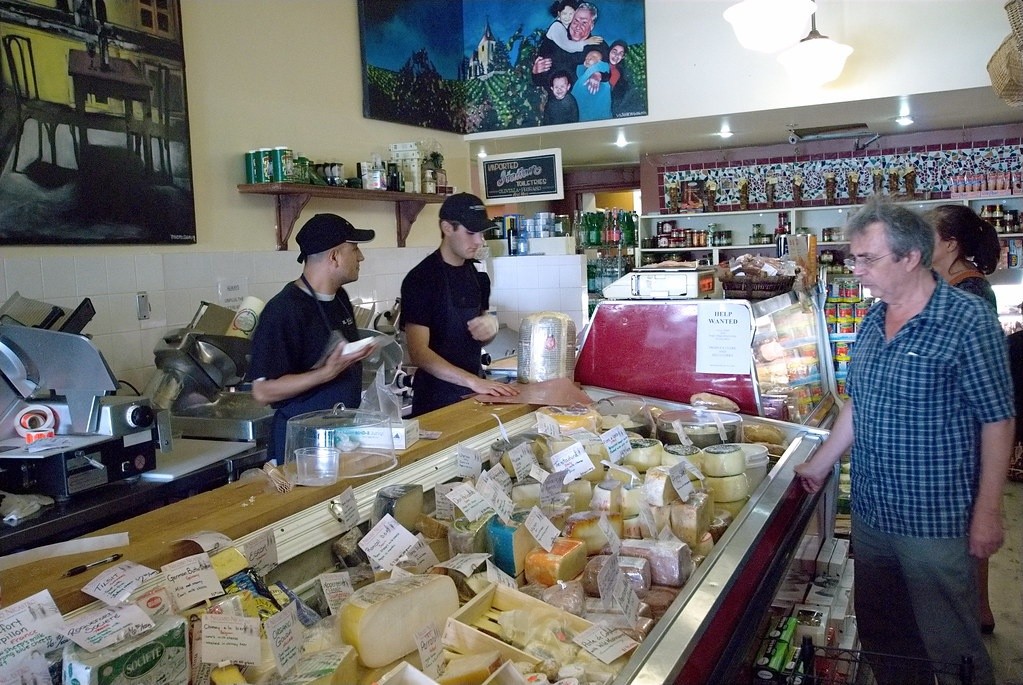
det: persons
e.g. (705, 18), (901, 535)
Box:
(923, 205), (1000, 634)
(399, 193), (520, 417)
(793, 200), (1016, 685)
(249, 213), (380, 467)
(532, 0), (633, 126)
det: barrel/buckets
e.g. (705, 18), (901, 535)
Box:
(724, 442), (769, 494)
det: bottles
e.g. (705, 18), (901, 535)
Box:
(794, 226), (857, 274)
(981, 205), (1023, 233)
(485, 208), (638, 295)
(950, 172), (1023, 191)
(645, 211), (791, 267)
(802, 635), (813, 685)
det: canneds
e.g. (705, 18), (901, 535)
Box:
(980, 203), (1023, 234)
(819, 249), (853, 274)
(821, 228), (831, 242)
(244, 145), (296, 184)
(644, 220), (732, 246)
(774, 212), (791, 243)
(796, 226), (809, 234)
(748, 224), (772, 245)
(779, 279), (868, 419)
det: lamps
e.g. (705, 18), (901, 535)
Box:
(722, 0), (857, 90)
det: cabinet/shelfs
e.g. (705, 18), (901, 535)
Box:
(638, 192), (1023, 279)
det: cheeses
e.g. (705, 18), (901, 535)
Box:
(185, 405), (750, 685)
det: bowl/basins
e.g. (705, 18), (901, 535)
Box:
(606, 431), (749, 519)
(295, 446), (340, 487)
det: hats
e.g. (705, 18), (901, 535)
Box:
(439, 192), (500, 233)
(295, 213), (375, 263)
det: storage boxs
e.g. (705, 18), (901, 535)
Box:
(772, 535), (857, 654)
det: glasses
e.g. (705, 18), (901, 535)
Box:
(844, 250), (897, 271)
